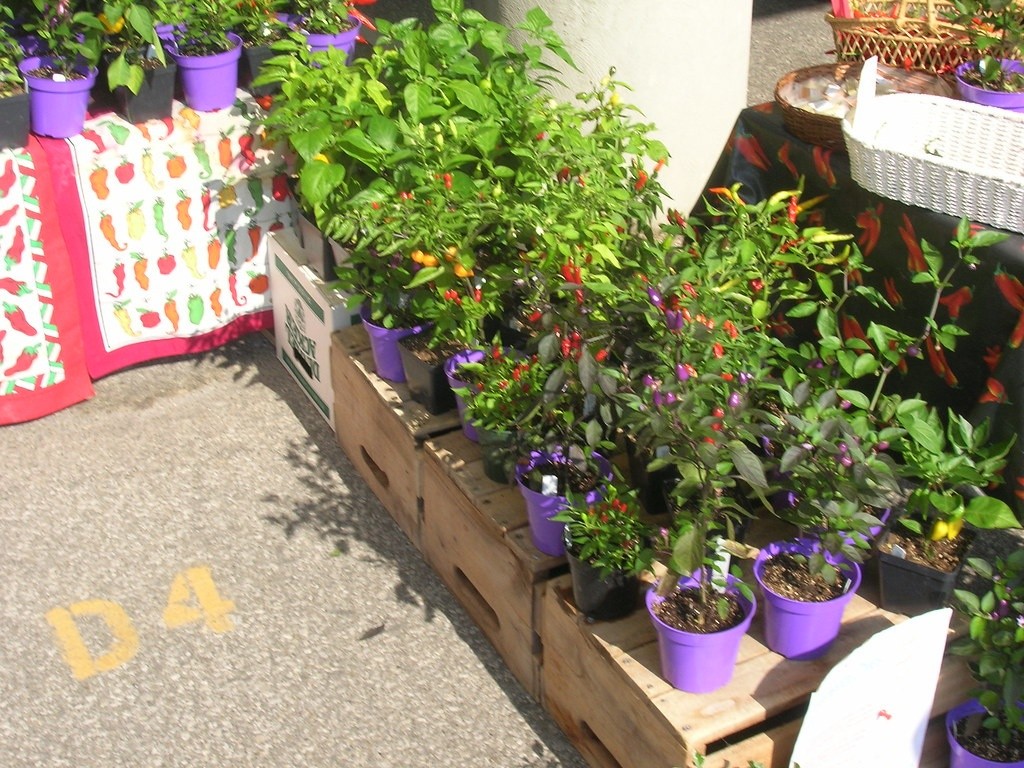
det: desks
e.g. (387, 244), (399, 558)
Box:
(684, 101), (1024, 523)
(0, 76), (305, 426)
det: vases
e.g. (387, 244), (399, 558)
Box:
(102, 48), (177, 123)
(288, 13), (362, 68)
(361, 299), (433, 382)
(19, 56), (100, 138)
(953, 57), (1024, 113)
(752, 537), (862, 660)
(299, 212), (339, 281)
(327, 235), (365, 293)
(558, 521), (652, 621)
(474, 412), (557, 483)
(946, 697), (1024, 768)
(167, 31), (242, 112)
(873, 514), (976, 617)
(443, 346), (530, 443)
(647, 567), (757, 695)
(395, 327), (456, 417)
(515, 444), (614, 555)
(238, 45), (304, 97)
(285, 178), (304, 248)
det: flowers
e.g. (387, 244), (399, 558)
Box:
(0, 0), (1024, 745)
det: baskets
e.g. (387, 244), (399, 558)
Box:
(841, 92), (1024, 234)
(774, 61), (961, 152)
(824, 0), (1024, 82)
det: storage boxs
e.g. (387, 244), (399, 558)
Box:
(326, 323), (462, 549)
(538, 573), (910, 768)
(266, 228), (410, 433)
(417, 429), (569, 701)
(703, 654), (984, 768)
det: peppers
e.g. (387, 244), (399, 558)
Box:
(931, 504), (1017, 623)
(372, 131), (919, 548)
(38, 0), (379, 48)
